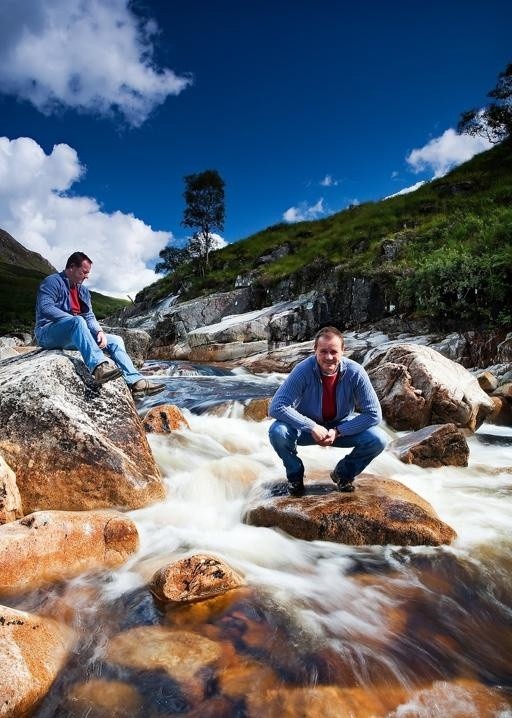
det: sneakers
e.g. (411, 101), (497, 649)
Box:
(93, 362), (123, 385)
(288, 477), (304, 495)
(132, 378), (167, 402)
(330, 469), (355, 492)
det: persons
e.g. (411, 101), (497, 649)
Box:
(268, 325), (387, 496)
(33, 252), (166, 400)
